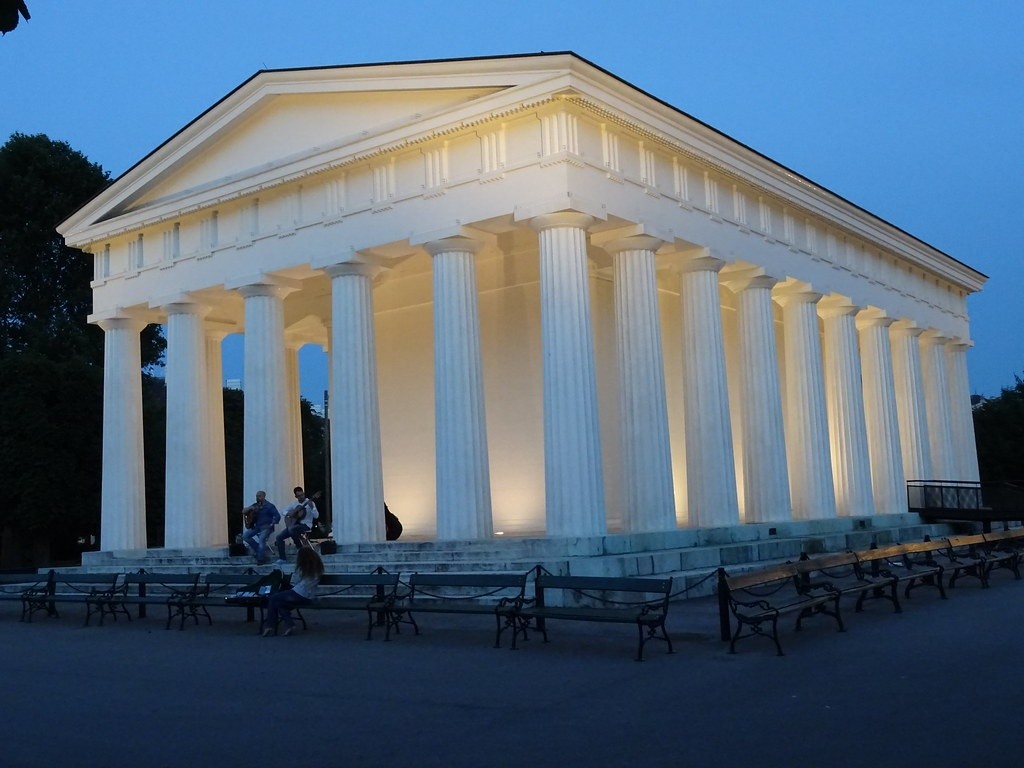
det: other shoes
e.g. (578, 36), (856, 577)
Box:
(257, 560), (264, 565)
(254, 554), (257, 558)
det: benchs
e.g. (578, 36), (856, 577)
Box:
(718, 527), (1024, 656)
(178, 567), (401, 641)
(383, 571), (532, 649)
(509, 573), (678, 663)
(0, 568), (202, 631)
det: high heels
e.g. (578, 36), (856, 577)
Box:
(281, 625), (296, 637)
(262, 627), (273, 637)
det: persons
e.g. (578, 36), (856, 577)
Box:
(242, 491), (281, 564)
(384, 502), (403, 541)
(276, 487), (320, 565)
(262, 547), (324, 637)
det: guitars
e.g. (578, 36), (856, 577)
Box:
(283, 490), (324, 530)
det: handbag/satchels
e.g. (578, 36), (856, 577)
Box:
(321, 536), (337, 555)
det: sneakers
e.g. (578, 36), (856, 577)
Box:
(272, 559), (287, 566)
(297, 546), (303, 553)
